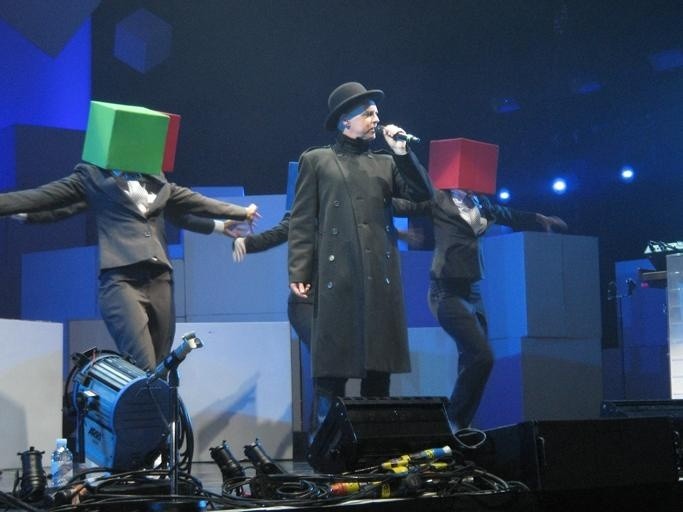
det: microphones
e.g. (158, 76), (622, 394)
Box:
(376, 124), (421, 144)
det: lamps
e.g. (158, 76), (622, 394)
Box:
(209, 391), (457, 491)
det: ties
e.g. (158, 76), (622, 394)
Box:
(466, 197), (481, 233)
(125, 172), (148, 215)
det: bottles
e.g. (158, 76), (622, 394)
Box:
(51, 438), (75, 489)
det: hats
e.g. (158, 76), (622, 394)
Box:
(324, 81), (385, 132)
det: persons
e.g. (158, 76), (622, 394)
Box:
(230, 212), (425, 357)
(286, 80), (434, 433)
(0, 163), (264, 384)
(9, 201), (248, 348)
(388, 189), (569, 427)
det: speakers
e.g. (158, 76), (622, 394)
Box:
(304, 393), (457, 475)
(454, 414), (679, 491)
